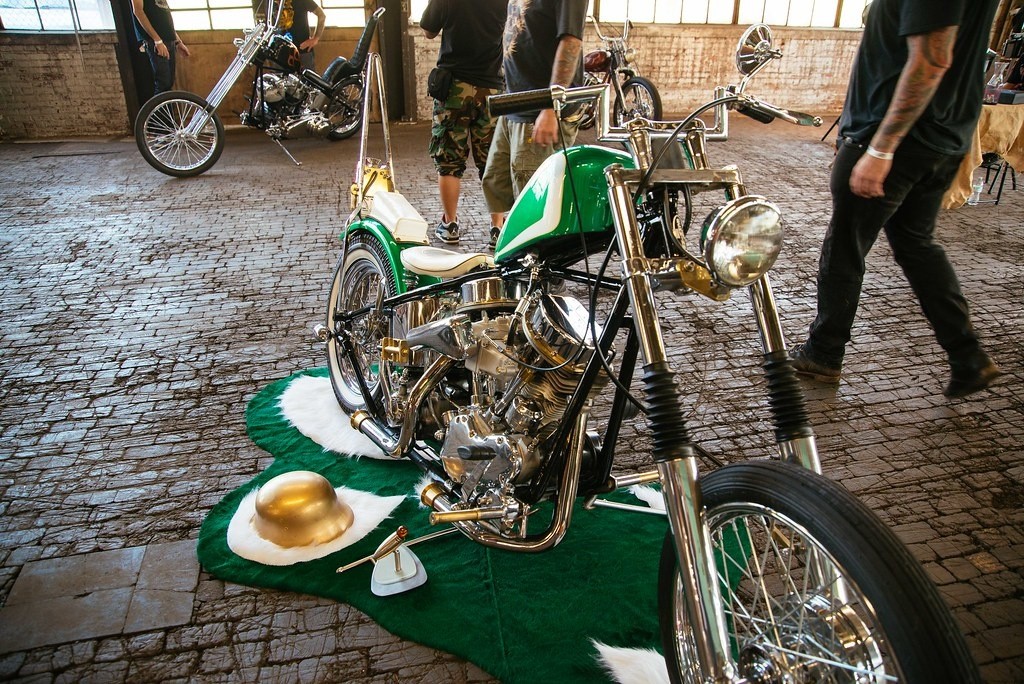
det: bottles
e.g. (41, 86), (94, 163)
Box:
(969, 177), (984, 206)
(983, 62), (1009, 105)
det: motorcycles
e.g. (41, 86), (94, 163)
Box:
(135, 0), (387, 179)
(553, 14), (664, 151)
(316, 25), (984, 684)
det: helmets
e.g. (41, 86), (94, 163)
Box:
(249, 471), (354, 549)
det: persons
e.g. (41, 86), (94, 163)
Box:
(264, 0), (326, 73)
(482, 0), (590, 214)
(419, 0), (508, 250)
(788, 0), (1005, 399)
(129, 0), (190, 149)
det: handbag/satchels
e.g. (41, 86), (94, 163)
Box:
(427, 68), (452, 101)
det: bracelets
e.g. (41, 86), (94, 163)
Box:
(865, 144), (894, 161)
(175, 40), (182, 45)
(154, 39), (162, 45)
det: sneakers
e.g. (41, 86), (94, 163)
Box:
(943, 348), (1001, 398)
(488, 217), (506, 249)
(435, 213), (460, 244)
(786, 344), (841, 383)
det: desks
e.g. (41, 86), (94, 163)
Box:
(942, 102), (1024, 209)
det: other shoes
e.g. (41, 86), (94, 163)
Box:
(154, 131), (192, 148)
